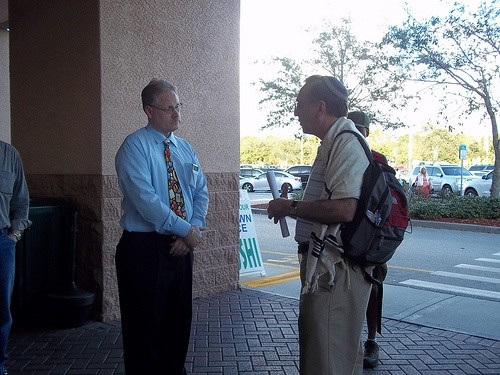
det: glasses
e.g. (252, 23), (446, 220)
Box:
(147, 102), (182, 114)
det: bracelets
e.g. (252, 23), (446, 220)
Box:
(183, 226), (195, 240)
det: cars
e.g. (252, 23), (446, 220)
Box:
(387, 160), (494, 199)
(239, 165), (314, 192)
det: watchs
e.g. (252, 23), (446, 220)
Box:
(289, 200), (299, 216)
(12, 231), (21, 241)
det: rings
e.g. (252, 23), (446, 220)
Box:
(182, 247), (186, 249)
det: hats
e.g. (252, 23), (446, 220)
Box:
(348, 111), (369, 129)
(322, 76), (348, 102)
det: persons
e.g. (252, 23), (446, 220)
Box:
(0, 140), (32, 375)
(268, 76), (434, 375)
(114, 78), (208, 375)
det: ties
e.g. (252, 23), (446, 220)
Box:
(163, 138), (188, 221)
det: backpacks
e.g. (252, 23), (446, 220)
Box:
(326, 130), (412, 266)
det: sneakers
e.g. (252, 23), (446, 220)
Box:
(362, 340), (379, 367)
